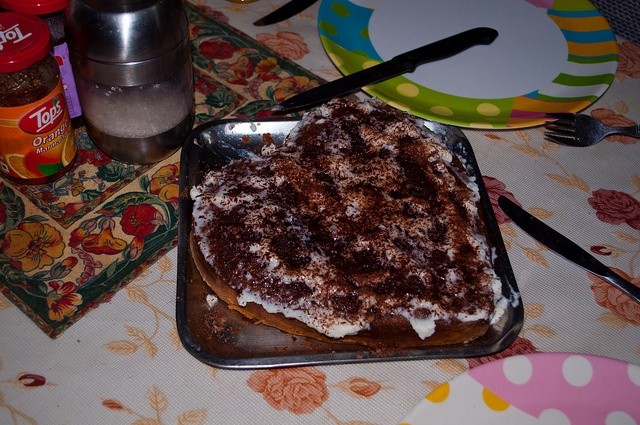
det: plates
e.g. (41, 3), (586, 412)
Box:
(175, 118), (525, 370)
(316, 1), (619, 133)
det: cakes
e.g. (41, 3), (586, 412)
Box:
(191, 96), (510, 340)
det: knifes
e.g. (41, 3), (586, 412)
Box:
(272, 26), (499, 113)
(497, 194), (640, 305)
(253, 0), (323, 26)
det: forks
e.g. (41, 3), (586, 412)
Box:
(539, 112), (637, 148)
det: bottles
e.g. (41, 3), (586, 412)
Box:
(79, 0), (195, 166)
